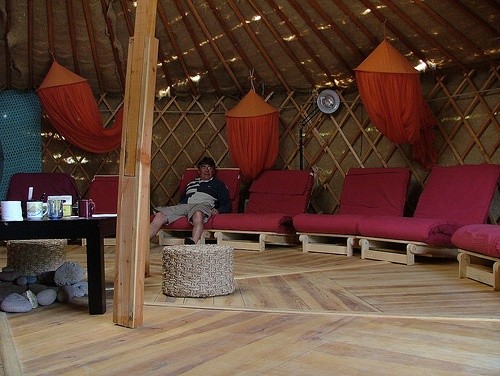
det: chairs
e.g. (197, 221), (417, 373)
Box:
(450, 223), (500, 292)
(357, 163), (500, 266)
(151, 168), (240, 246)
(212, 169), (314, 252)
(292, 167), (413, 256)
(7, 172), (120, 246)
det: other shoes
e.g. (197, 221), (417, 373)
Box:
(184, 237), (195, 245)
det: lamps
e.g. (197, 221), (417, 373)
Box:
(299, 88), (341, 170)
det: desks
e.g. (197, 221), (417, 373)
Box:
(0, 215), (116, 315)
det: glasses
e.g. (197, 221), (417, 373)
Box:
(199, 166), (214, 169)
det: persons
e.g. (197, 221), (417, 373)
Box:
(148, 157), (232, 245)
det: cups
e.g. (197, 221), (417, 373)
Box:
(46, 199), (63, 218)
(26, 201), (48, 219)
(0, 201), (22, 220)
(22, 200), (36, 216)
(78, 199), (95, 217)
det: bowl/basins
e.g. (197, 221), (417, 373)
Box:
(72, 207), (79, 215)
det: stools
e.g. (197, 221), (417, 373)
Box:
(161, 243), (236, 297)
(7, 239), (68, 278)
